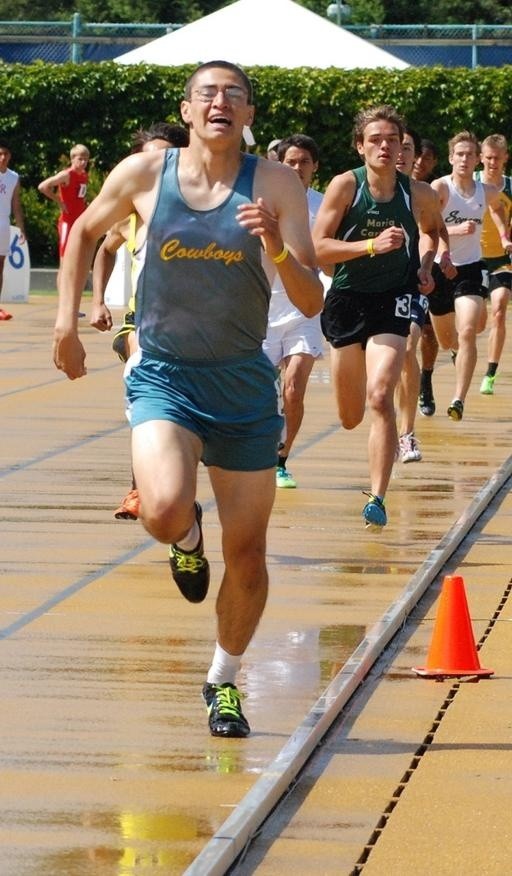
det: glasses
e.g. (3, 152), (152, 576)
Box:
(185, 85), (249, 103)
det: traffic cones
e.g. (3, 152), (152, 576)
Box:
(418, 574), (494, 682)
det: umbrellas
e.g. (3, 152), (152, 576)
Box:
(112, 0), (413, 72)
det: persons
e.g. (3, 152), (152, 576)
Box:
(37, 144), (92, 318)
(312, 105), (511, 535)
(51, 59), (326, 737)
(262, 133), (333, 490)
(87, 121), (191, 522)
(0, 141), (27, 321)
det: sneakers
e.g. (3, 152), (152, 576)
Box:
(201, 680), (251, 739)
(400, 429), (422, 463)
(418, 383), (436, 416)
(113, 488), (141, 521)
(481, 375), (496, 393)
(451, 349), (457, 366)
(447, 399), (464, 420)
(362, 490), (387, 534)
(0, 308), (12, 320)
(168, 499), (210, 604)
(275, 465), (297, 488)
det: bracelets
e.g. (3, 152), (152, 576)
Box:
(273, 246), (290, 264)
